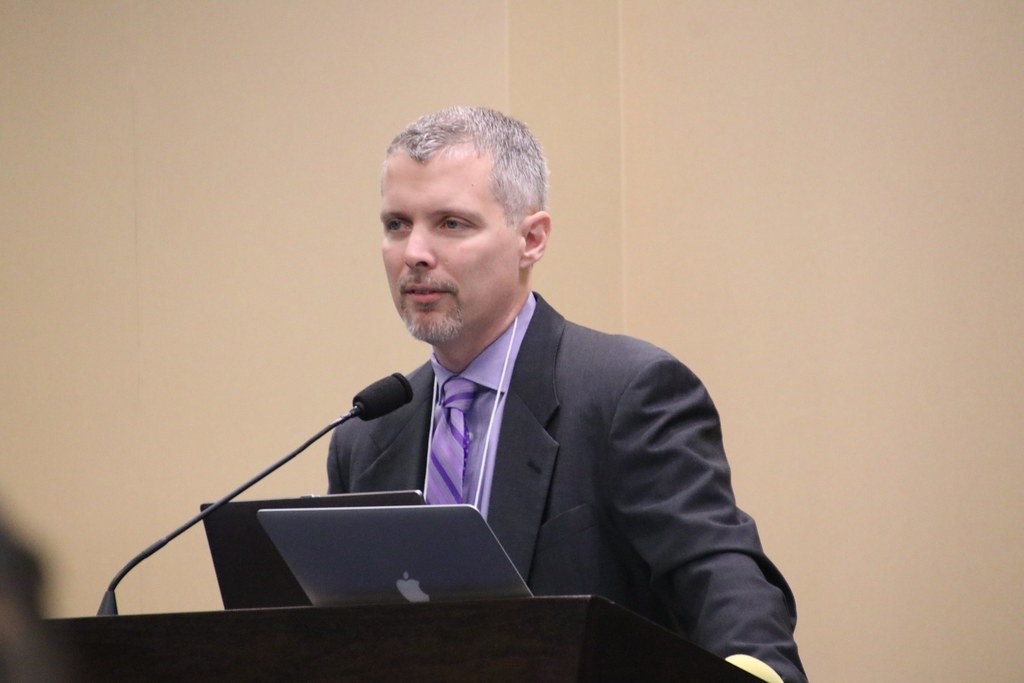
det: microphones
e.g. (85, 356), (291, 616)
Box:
(97, 373), (414, 617)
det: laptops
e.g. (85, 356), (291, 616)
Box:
(200, 490), (534, 610)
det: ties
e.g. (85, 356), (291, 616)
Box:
(425, 377), (478, 505)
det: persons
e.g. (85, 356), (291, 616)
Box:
(327, 104), (808, 683)
